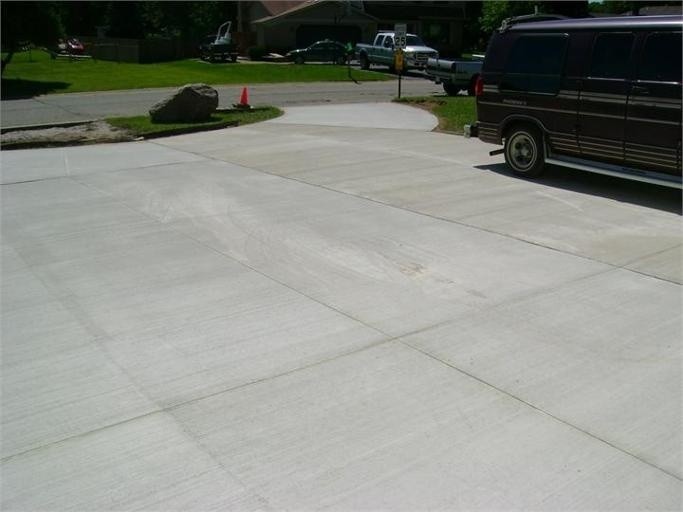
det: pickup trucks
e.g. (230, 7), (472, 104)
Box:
(422, 54), (485, 98)
(352, 31), (439, 76)
(199, 34), (240, 65)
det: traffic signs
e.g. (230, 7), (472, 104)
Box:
(392, 23), (407, 52)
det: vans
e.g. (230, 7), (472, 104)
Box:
(462, 3), (683, 196)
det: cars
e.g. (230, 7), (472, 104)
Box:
(283, 39), (354, 67)
(64, 37), (84, 55)
(44, 38), (69, 54)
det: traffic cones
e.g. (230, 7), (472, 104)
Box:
(239, 87), (248, 104)
